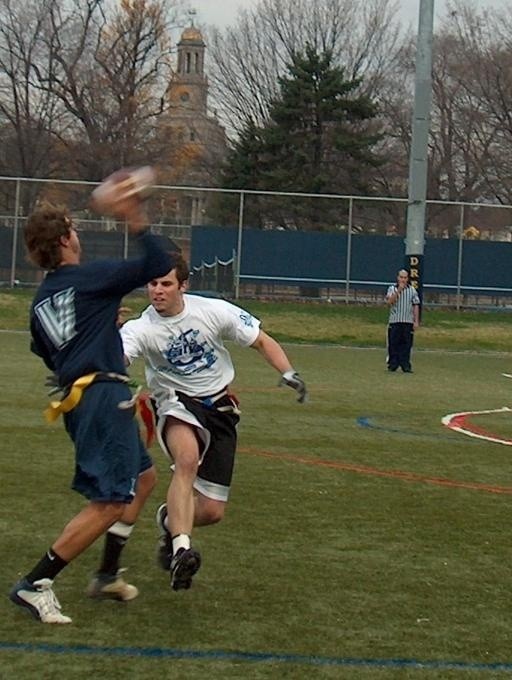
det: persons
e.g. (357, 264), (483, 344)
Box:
(43, 251), (307, 592)
(383, 268), (423, 375)
(8, 189), (184, 631)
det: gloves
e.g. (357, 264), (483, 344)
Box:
(277, 369), (308, 404)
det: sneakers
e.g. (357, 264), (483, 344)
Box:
(383, 367), (398, 372)
(8, 578), (73, 624)
(169, 547), (202, 591)
(403, 368), (415, 373)
(83, 577), (140, 602)
(156, 502), (174, 571)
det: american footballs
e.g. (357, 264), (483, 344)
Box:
(90, 165), (160, 214)
